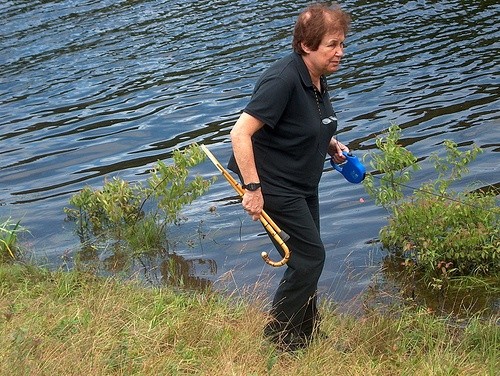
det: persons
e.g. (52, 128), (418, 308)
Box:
(227, 8), (353, 352)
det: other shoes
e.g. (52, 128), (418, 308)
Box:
(263, 329), (329, 353)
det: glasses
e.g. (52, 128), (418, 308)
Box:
(318, 114), (339, 159)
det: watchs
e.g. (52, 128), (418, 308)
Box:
(242, 183), (262, 192)
